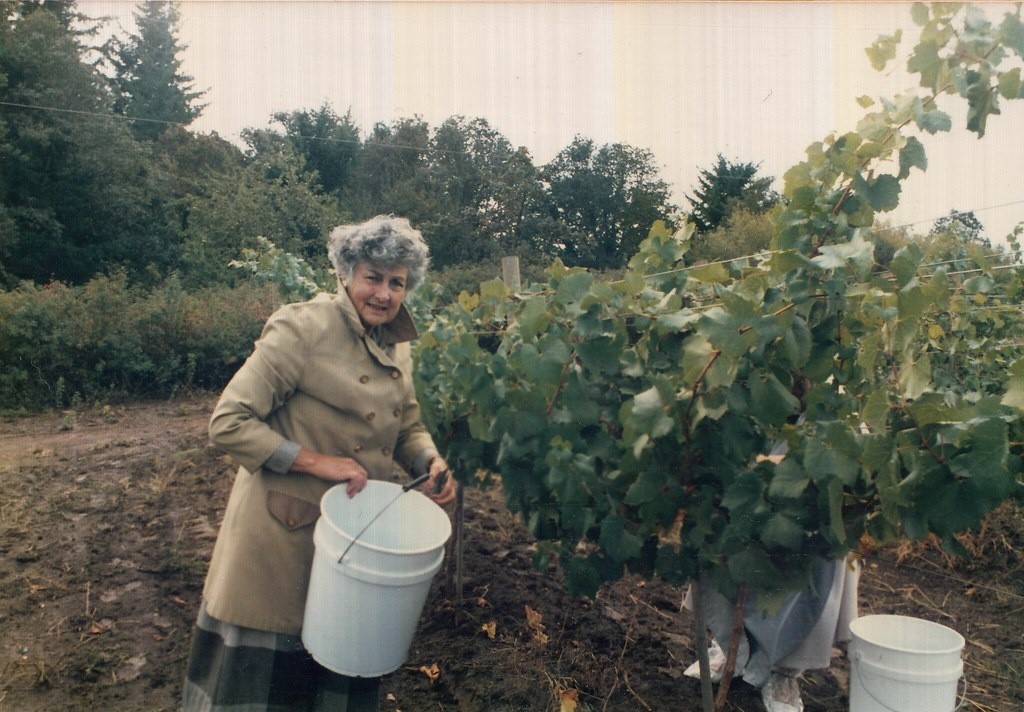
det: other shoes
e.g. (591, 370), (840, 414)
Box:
(681, 632), (745, 684)
(762, 669), (805, 712)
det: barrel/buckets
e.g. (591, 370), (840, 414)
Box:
(846, 614), (966, 712)
(299, 478), (453, 679)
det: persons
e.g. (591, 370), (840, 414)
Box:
(177, 212), (459, 711)
(679, 368), (869, 711)
